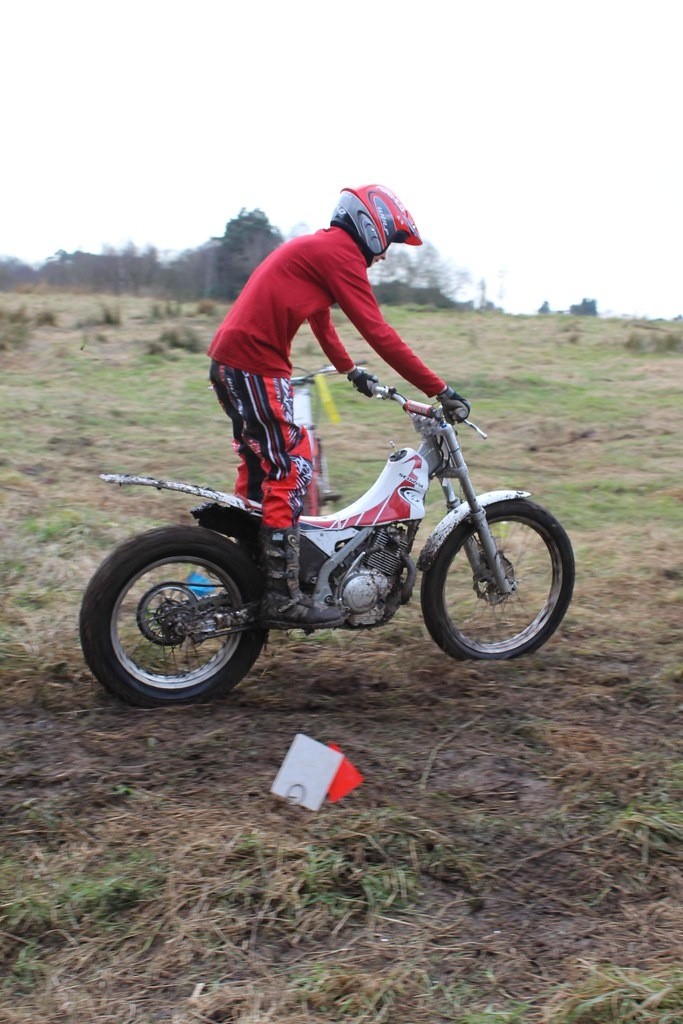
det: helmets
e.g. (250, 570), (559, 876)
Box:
(330, 184), (423, 255)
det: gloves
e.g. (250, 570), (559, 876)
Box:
(347, 367), (379, 398)
(436, 385), (471, 426)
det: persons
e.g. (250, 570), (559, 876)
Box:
(206, 184), (472, 632)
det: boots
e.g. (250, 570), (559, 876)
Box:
(259, 524), (344, 630)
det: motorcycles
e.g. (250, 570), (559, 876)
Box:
(71, 372), (577, 706)
(207, 358), (370, 517)
